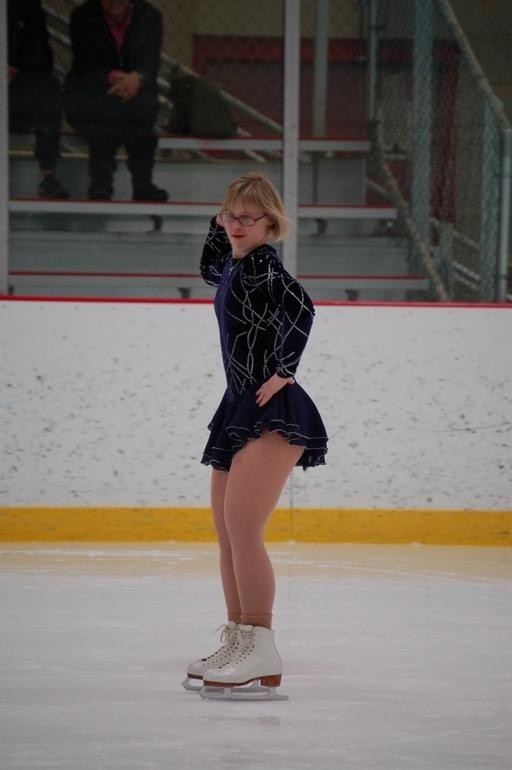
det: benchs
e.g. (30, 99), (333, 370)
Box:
(7, 133), (429, 302)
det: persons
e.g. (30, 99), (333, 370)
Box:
(6, 1), (71, 198)
(183, 172), (331, 691)
(62, 0), (170, 204)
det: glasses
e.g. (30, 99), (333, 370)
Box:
(219, 212), (268, 226)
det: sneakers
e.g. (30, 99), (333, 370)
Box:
(38, 172), (168, 201)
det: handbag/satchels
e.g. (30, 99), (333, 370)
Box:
(161, 75), (235, 137)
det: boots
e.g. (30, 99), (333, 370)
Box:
(187, 621), (282, 687)
